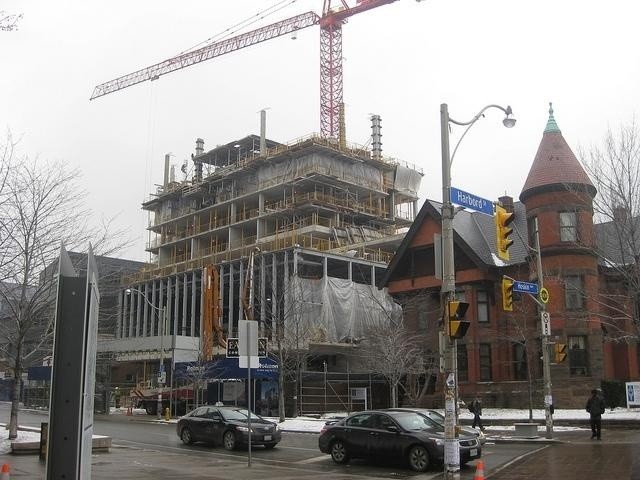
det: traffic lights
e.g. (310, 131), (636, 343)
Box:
(496, 208), (516, 256)
(502, 277), (515, 312)
(555, 343), (568, 364)
(446, 298), (470, 340)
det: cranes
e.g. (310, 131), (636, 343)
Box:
(81, 0), (423, 149)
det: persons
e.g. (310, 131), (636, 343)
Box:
(471, 394), (485, 431)
(586, 389), (605, 441)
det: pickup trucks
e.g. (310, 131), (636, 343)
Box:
(140, 395), (193, 417)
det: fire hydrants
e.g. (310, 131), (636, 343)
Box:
(164, 407), (171, 422)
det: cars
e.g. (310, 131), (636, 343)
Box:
(176, 404), (283, 452)
(357, 407), (487, 445)
(316, 407), (483, 472)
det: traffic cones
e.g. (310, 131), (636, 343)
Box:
(125, 407), (133, 416)
(472, 460), (487, 480)
(0, 463), (12, 479)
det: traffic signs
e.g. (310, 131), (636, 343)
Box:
(224, 337), (272, 362)
(513, 280), (539, 294)
(449, 186), (493, 216)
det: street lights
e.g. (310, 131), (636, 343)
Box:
(125, 287), (166, 419)
(434, 96), (521, 478)
